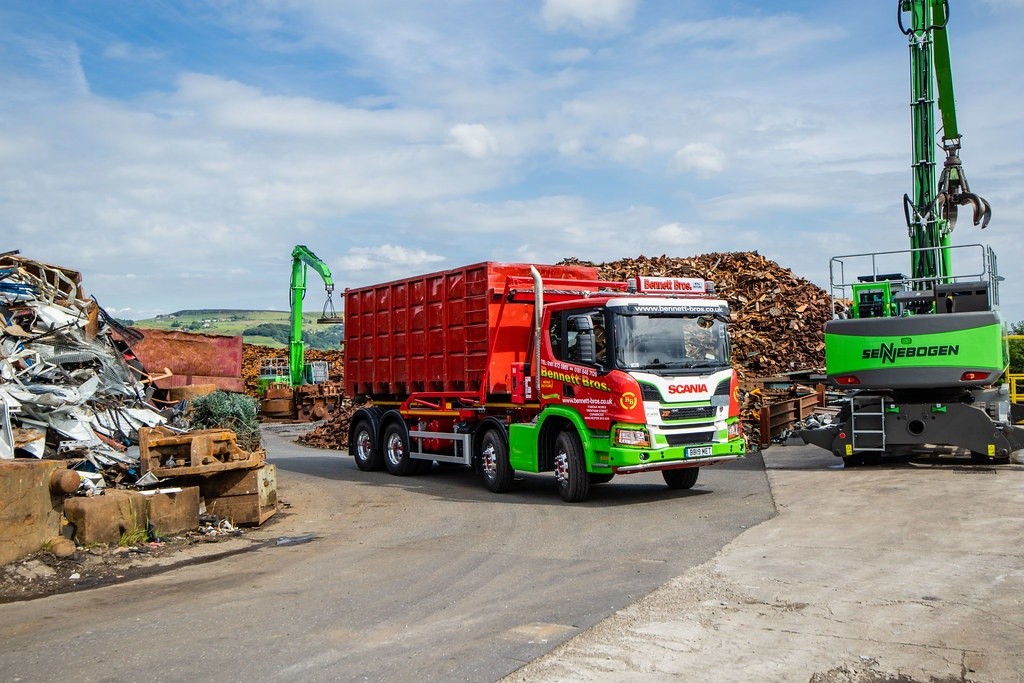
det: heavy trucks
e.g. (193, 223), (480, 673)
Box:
(343, 260), (748, 503)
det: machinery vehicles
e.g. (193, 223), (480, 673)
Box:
(254, 245), (343, 397)
(798, 0), (1024, 467)
